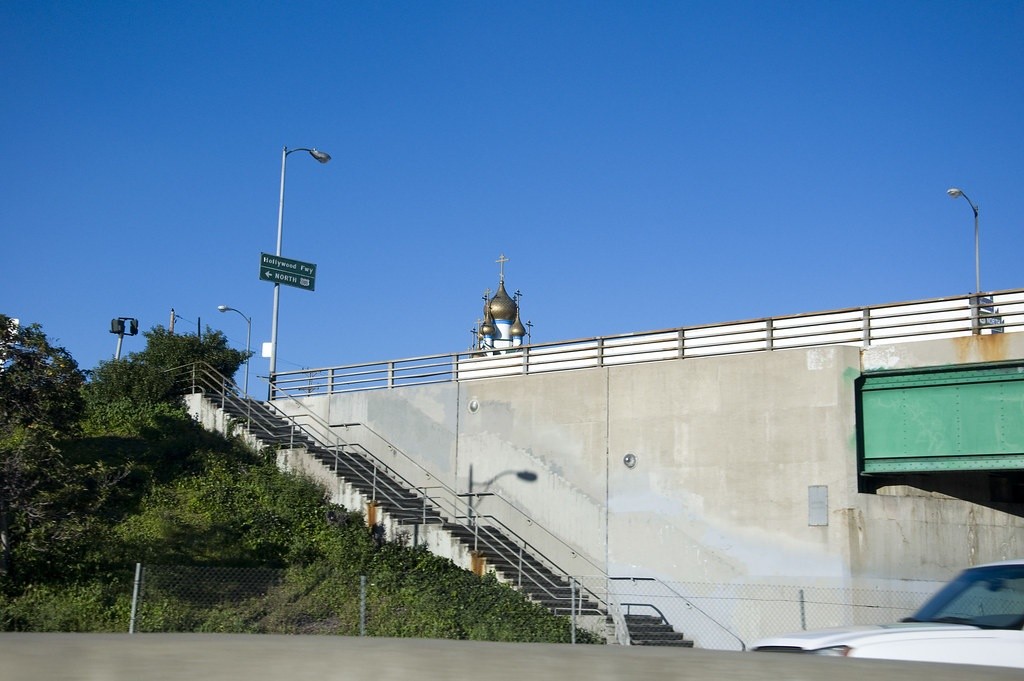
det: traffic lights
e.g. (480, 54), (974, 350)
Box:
(110, 319), (122, 335)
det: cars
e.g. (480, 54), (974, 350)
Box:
(738, 555), (1024, 671)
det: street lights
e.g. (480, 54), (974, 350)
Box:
(266, 145), (333, 394)
(947, 188), (982, 337)
(217, 305), (253, 400)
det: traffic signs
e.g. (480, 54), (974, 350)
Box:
(258, 265), (316, 292)
